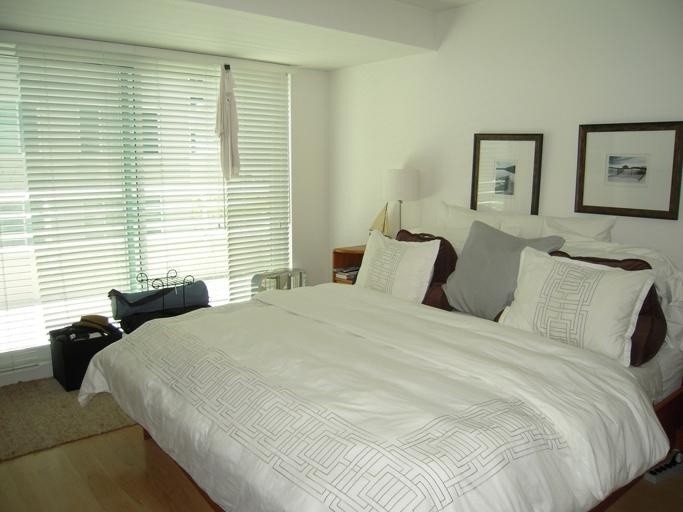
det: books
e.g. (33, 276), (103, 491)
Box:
(336, 268), (357, 280)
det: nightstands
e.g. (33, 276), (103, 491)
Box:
(332, 245), (368, 286)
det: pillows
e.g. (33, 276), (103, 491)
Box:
(443, 221), (567, 321)
(355, 229), (442, 307)
(501, 246), (658, 369)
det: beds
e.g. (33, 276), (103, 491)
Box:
(103, 204), (683, 511)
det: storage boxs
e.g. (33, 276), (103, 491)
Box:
(48, 324), (122, 393)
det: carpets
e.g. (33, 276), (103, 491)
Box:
(1, 375), (139, 465)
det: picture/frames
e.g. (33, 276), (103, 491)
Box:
(574, 121), (683, 220)
(470, 133), (542, 215)
